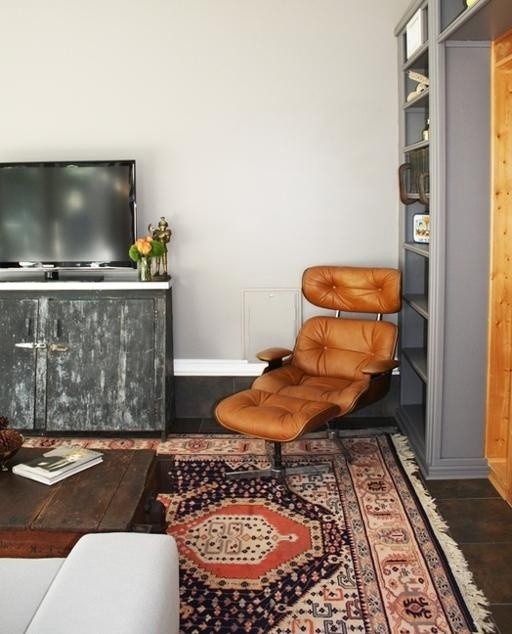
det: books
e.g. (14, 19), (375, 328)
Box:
(11, 443), (103, 486)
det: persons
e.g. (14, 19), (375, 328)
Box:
(150, 216), (172, 277)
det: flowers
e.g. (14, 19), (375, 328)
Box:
(129, 237), (164, 280)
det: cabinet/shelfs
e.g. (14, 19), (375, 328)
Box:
(394, 0), (512, 480)
(0, 286), (176, 441)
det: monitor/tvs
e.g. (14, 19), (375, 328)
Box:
(0, 159), (138, 283)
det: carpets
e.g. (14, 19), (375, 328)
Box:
(22, 426), (497, 634)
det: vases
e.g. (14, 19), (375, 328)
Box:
(137, 257), (152, 282)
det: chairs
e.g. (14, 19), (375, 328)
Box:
(0, 532), (179, 634)
(215, 266), (402, 499)
